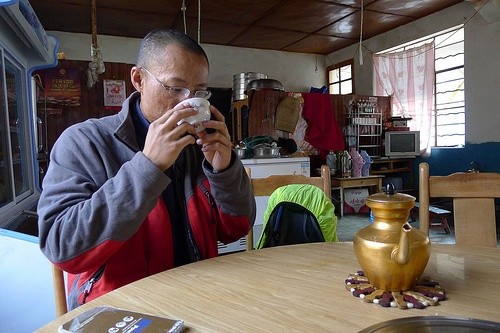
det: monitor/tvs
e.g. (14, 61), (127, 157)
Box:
(385, 131), (420, 159)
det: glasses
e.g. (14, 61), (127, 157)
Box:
(139, 66), (213, 99)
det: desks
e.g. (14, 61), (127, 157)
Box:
(31, 241), (500, 333)
(329, 175), (384, 217)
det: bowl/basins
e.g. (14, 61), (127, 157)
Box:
(173, 98), (211, 134)
(253, 146), (279, 158)
(244, 79), (286, 95)
(232, 149), (246, 159)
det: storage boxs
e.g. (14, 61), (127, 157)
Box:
(343, 188), (370, 215)
(393, 121), (410, 131)
(381, 177), (403, 190)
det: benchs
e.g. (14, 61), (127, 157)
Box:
(394, 188), (452, 235)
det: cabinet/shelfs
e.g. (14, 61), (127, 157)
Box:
(346, 102), (382, 159)
(369, 155), (417, 188)
(230, 100), (249, 143)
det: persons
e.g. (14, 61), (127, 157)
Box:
(37, 26), (256, 312)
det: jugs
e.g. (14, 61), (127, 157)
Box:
(326, 148), (371, 179)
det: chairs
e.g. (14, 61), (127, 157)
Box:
(418, 162), (500, 245)
(245, 165), (332, 252)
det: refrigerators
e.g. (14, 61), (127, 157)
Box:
(217, 157), (310, 254)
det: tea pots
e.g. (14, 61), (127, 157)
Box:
(353, 182), (432, 292)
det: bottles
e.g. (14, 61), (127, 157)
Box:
(357, 99), (377, 112)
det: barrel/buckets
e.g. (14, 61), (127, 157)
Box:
(232, 72), (268, 102)
